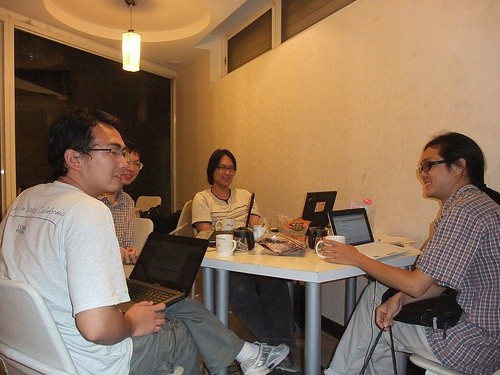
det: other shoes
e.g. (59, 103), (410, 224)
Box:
(273, 360), (301, 375)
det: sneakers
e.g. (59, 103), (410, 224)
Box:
(240, 341), (290, 375)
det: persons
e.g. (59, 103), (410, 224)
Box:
(321, 133), (500, 375)
(96, 142), (141, 264)
(1, 107), (289, 374)
(192, 148), (303, 375)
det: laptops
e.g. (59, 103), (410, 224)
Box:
(327, 208), (410, 259)
(115, 232), (210, 315)
(271, 191), (339, 232)
(195, 192), (255, 247)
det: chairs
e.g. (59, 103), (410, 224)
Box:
(125, 194), (196, 272)
(0, 280), (76, 375)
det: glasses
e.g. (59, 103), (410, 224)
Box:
(215, 166), (237, 173)
(124, 160), (143, 170)
(419, 159), (457, 173)
(78, 148), (127, 160)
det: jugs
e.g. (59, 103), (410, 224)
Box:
(233, 226), (254, 252)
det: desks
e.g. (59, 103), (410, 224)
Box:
(200, 245), (422, 375)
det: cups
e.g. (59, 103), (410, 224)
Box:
(316, 235), (346, 259)
(253, 224), (266, 241)
(309, 226), (327, 249)
(216, 234), (237, 256)
(214, 218), (236, 231)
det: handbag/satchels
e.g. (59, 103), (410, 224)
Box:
(380, 287), (461, 330)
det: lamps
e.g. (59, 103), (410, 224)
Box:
(122, 0), (143, 72)
